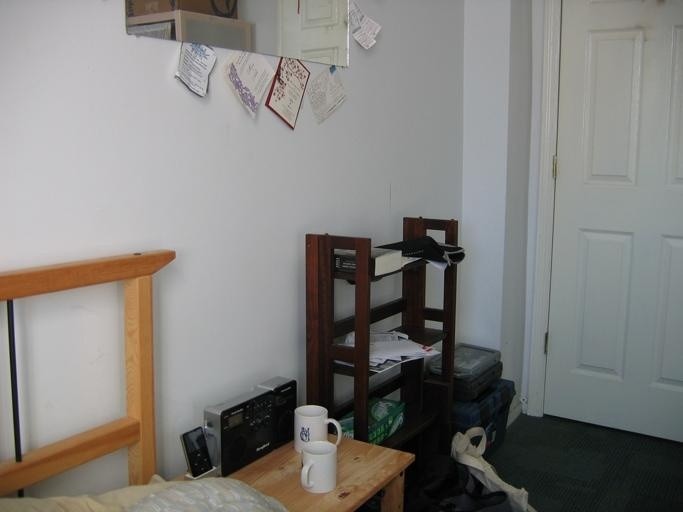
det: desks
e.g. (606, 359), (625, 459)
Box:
(226, 433), (417, 512)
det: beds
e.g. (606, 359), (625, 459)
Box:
(0, 247), (286, 512)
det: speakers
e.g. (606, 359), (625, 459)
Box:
(257, 376), (299, 449)
(204, 398), (249, 477)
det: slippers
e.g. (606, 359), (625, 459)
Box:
(417, 470), (510, 511)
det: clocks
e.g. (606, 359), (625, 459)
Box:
(211, 1), (237, 18)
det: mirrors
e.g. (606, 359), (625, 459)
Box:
(123, 0), (350, 70)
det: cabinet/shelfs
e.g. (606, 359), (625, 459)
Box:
(125, 9), (253, 53)
(302, 218), (458, 511)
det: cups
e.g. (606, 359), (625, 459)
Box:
(298, 440), (337, 493)
(293, 404), (341, 453)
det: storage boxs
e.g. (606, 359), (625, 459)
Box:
(427, 341), (504, 398)
(129, 1), (239, 21)
(448, 376), (517, 454)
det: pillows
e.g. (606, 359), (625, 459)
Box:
(93, 476), (287, 512)
(0, 489), (109, 512)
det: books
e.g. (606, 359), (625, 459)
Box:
(333, 330), (440, 373)
(333, 247), (402, 278)
